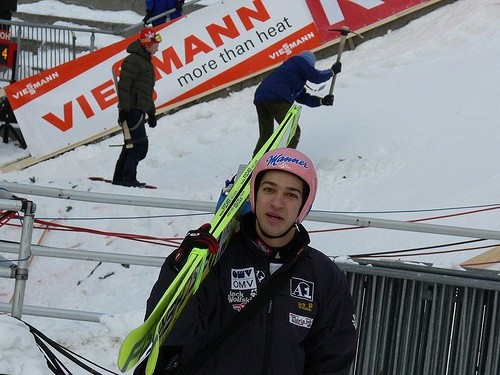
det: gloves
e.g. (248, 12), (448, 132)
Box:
(148, 115), (157, 128)
(175, 0), (183, 12)
(175, 223), (220, 270)
(322, 95), (334, 106)
(118, 109), (129, 124)
(331, 61), (341, 74)
(142, 12), (151, 25)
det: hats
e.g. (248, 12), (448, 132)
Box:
(299, 51), (316, 67)
(140, 28), (157, 46)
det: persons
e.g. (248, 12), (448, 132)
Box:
(0, 0), (18, 32)
(143, 0), (186, 29)
(133, 148), (358, 375)
(111, 28), (162, 186)
(253, 51), (342, 161)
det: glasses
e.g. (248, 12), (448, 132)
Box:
(155, 34), (162, 43)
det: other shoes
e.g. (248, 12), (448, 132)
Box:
(113, 176), (145, 187)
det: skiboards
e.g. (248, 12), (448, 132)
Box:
(116, 100), (303, 375)
(88, 177), (158, 189)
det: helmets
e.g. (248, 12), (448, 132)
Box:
(250, 147), (317, 224)
